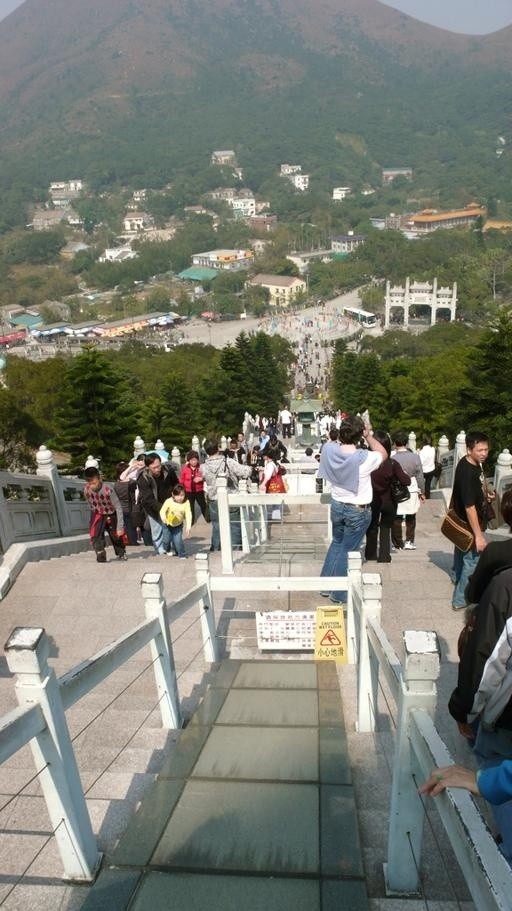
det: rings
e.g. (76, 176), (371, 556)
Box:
(435, 774), (442, 782)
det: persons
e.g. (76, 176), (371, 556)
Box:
(263, 300), (336, 399)
(447, 569), (511, 756)
(315, 401), (511, 610)
(83, 405), (298, 562)
(415, 758), (512, 871)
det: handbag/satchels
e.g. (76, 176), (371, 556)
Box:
(128, 510), (146, 528)
(262, 463), (286, 494)
(203, 495), (211, 524)
(440, 506), (478, 554)
(390, 457), (411, 503)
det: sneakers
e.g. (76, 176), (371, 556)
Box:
(452, 600), (469, 611)
(116, 553), (128, 561)
(403, 540), (417, 550)
(179, 556), (187, 560)
(328, 596), (348, 604)
(451, 579), (458, 585)
(319, 591), (330, 597)
(392, 543), (402, 552)
(166, 551), (173, 557)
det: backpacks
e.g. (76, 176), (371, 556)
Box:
(434, 447), (443, 479)
(212, 456), (241, 514)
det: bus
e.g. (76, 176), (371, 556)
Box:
(342, 305), (377, 328)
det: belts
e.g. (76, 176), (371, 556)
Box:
(340, 502), (371, 509)
